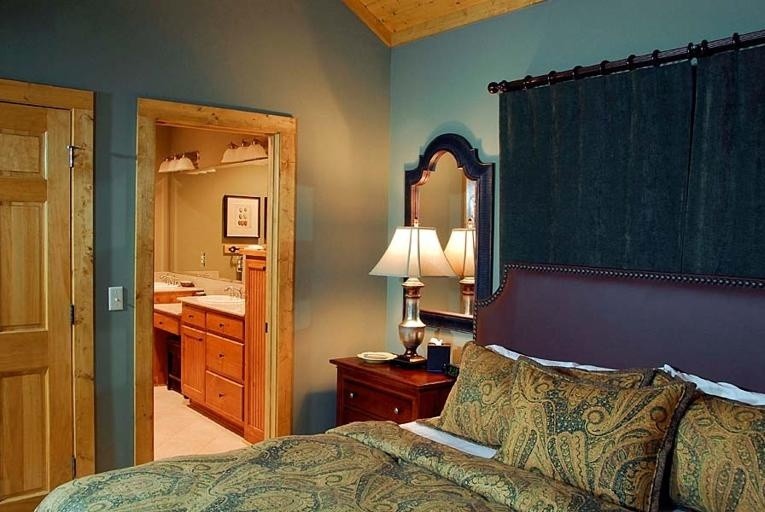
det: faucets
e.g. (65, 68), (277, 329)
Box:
(160, 273), (173, 284)
(224, 286), (234, 296)
(235, 287), (243, 299)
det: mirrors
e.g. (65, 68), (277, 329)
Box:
(167, 164), (267, 285)
(404, 133), (495, 333)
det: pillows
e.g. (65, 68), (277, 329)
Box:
(496, 356), (696, 511)
(419, 340), (514, 448)
(649, 369), (764, 511)
(547, 364), (652, 387)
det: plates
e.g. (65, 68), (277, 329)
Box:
(356, 351), (397, 364)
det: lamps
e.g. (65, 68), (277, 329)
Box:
(221, 137), (268, 166)
(157, 152), (201, 173)
(368, 217), (457, 371)
(444, 219), (476, 317)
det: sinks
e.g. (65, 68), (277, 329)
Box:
(178, 294), (241, 305)
(154, 281), (177, 288)
(208, 302), (244, 316)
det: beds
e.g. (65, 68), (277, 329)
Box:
(31, 264), (765, 512)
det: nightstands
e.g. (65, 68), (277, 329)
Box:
(330, 355), (456, 427)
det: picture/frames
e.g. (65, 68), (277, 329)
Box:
(223, 194), (261, 239)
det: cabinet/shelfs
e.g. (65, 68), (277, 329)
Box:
(153, 248), (266, 444)
